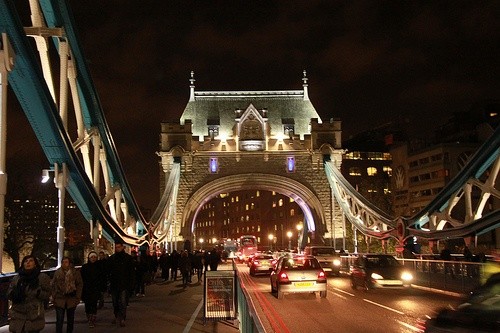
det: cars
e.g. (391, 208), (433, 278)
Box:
(424, 269), (500, 333)
(251, 254), (277, 276)
(271, 255), (327, 300)
(350, 253), (414, 293)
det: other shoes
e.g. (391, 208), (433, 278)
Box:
(97, 300), (105, 309)
(159, 273), (203, 284)
(112, 318), (131, 325)
(136, 292), (146, 297)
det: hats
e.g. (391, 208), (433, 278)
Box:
(89, 252), (98, 258)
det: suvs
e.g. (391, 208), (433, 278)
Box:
(305, 245), (343, 276)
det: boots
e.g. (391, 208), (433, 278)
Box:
(87, 313), (97, 327)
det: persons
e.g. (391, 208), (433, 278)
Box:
(339, 247), (345, 254)
(463, 245), (472, 262)
(439, 245), (456, 282)
(107, 239), (136, 328)
(94, 244), (228, 299)
(80, 250), (104, 330)
(49, 256), (84, 333)
(7, 253), (52, 333)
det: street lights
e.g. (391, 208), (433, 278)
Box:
(40, 169), (66, 268)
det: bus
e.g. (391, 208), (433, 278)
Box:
(239, 234), (257, 260)
